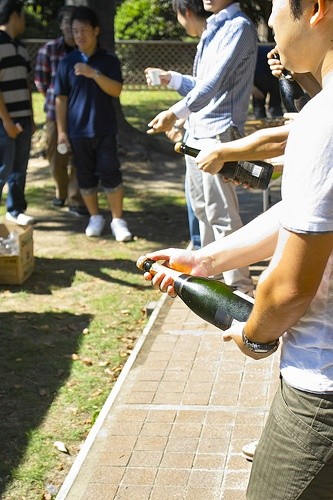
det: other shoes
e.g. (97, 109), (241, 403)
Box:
(52, 199), (63, 205)
(68, 206), (88, 217)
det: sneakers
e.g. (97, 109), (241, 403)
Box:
(5, 212), (33, 225)
(85, 215), (106, 237)
(110, 220), (132, 241)
(242, 441), (258, 462)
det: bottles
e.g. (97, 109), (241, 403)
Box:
(272, 53), (308, 113)
(174, 142), (274, 191)
(136, 255), (256, 332)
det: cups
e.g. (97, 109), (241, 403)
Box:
(15, 123), (23, 132)
(57, 143), (68, 155)
(148, 69), (162, 85)
(174, 130), (183, 142)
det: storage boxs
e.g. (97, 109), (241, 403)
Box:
(0, 223), (35, 285)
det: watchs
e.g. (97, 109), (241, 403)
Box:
(93, 70), (101, 80)
(241, 326), (279, 354)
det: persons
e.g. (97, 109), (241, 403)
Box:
(146, 0), (309, 461)
(144, 0), (333, 500)
(34, 5), (91, 218)
(53, 7), (133, 242)
(0, 0), (35, 226)
